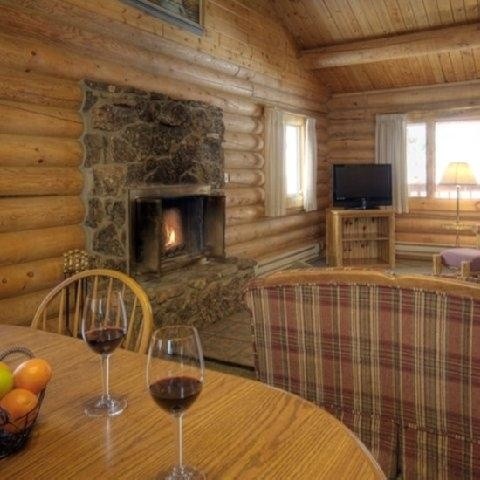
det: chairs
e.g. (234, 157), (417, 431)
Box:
(28, 269), (153, 353)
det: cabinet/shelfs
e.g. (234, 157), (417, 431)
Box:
(324, 208), (396, 268)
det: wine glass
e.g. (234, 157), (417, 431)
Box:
(147, 327), (205, 479)
(82, 291), (127, 418)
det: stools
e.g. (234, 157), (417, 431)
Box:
(433, 247), (480, 279)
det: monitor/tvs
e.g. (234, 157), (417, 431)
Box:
(333, 164), (392, 210)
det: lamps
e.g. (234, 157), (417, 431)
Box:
(436, 162), (480, 247)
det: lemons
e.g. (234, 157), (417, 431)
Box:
(0, 360), (13, 398)
